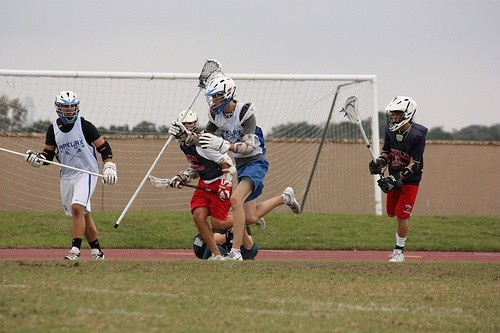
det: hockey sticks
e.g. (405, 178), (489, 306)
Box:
(147, 175), (219, 193)
(113, 59), (222, 229)
(0, 148), (104, 178)
(341, 95), (384, 183)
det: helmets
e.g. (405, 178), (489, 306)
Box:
(385, 96), (416, 132)
(203, 76), (237, 116)
(54, 90), (80, 127)
(177, 110), (198, 132)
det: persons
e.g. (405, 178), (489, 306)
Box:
(25, 90), (117, 260)
(202, 206), (258, 260)
(370, 96), (428, 262)
(170, 109), (265, 260)
(168, 77), (300, 261)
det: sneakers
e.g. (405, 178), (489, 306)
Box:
(207, 253), (224, 261)
(284, 187), (300, 214)
(223, 250), (243, 260)
(256, 216), (265, 231)
(90, 248), (104, 261)
(388, 249), (404, 262)
(65, 246), (81, 260)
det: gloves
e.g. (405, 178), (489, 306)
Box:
(168, 120), (197, 144)
(101, 161), (117, 185)
(24, 150), (46, 168)
(376, 173), (402, 194)
(218, 178), (232, 201)
(198, 133), (230, 154)
(369, 155), (388, 175)
(169, 171), (191, 190)
